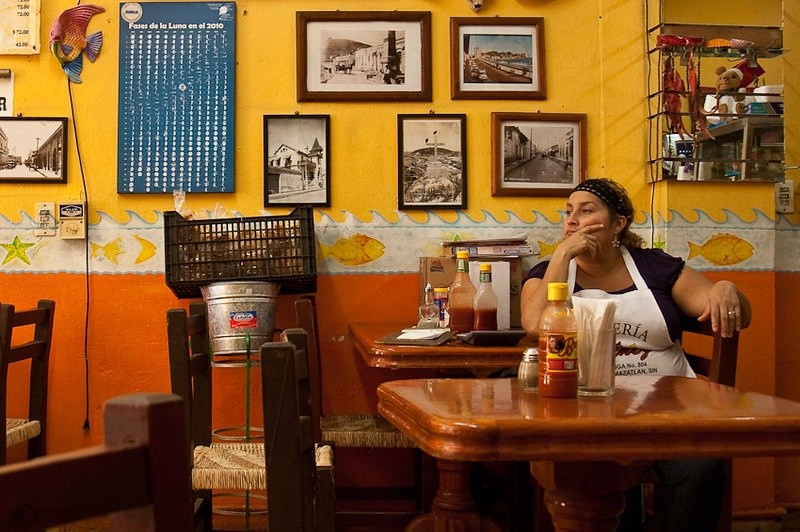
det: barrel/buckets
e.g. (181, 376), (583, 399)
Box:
(200, 281), (281, 355)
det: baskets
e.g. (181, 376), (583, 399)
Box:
(163, 205), (317, 299)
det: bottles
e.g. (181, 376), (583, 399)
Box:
(537, 282), (581, 399)
(517, 348), (539, 393)
(418, 284), (440, 320)
(447, 250), (477, 333)
(473, 264), (498, 331)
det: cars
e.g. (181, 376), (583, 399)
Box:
(466, 57), (489, 80)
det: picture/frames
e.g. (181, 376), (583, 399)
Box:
(261, 113), (331, 210)
(295, 9), (434, 103)
(448, 16), (549, 103)
(489, 111), (590, 198)
(0, 116), (69, 185)
(396, 112), (469, 212)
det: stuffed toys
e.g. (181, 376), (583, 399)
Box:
(714, 61), (751, 120)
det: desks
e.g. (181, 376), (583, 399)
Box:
(375, 374), (800, 532)
(347, 319), (544, 379)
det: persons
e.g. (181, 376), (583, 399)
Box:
(521, 178), (738, 532)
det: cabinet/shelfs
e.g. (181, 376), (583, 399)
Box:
(644, 21), (800, 185)
(208, 327), (286, 532)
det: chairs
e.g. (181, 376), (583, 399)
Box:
(165, 293), (425, 532)
(0, 299), (56, 466)
(650, 317), (741, 532)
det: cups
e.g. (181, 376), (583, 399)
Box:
(578, 331), (617, 397)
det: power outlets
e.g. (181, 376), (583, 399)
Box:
(774, 179), (794, 214)
(34, 201), (57, 237)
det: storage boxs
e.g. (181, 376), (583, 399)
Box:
(417, 255), (525, 329)
(162, 203), (319, 301)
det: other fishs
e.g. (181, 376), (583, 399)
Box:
(49, 4), (105, 84)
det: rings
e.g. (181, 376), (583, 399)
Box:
(727, 310), (736, 315)
